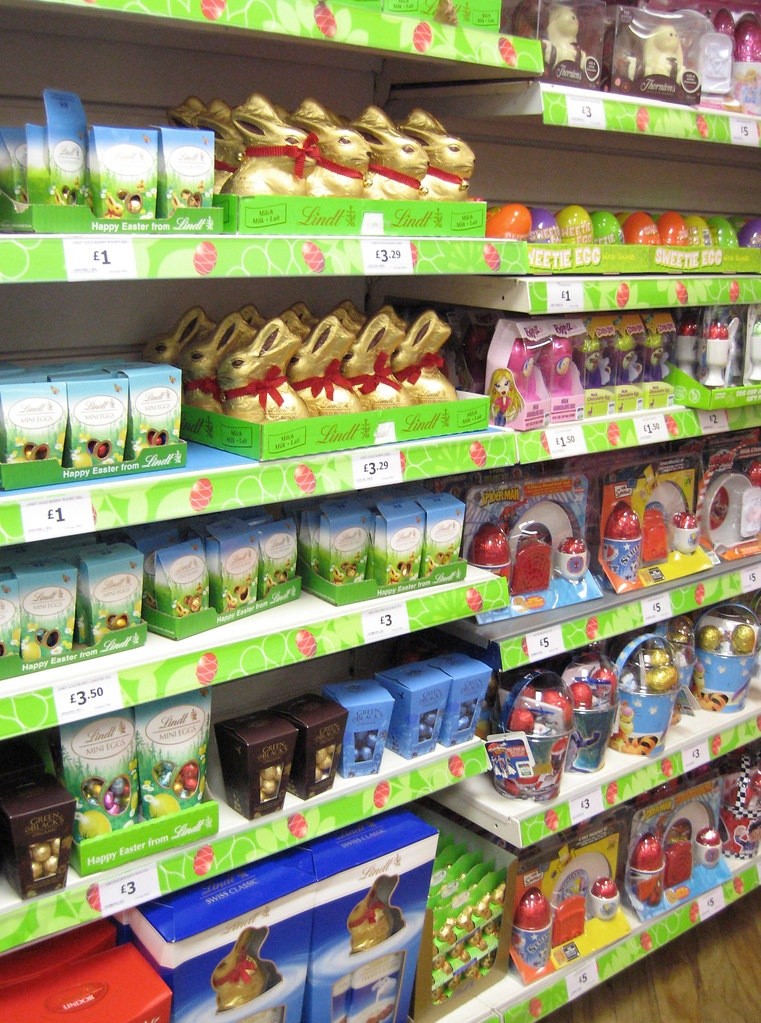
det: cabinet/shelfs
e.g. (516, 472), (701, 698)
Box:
(0, 0), (545, 1023)
(382, 79), (761, 1023)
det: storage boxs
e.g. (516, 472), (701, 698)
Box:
(529, 243), (761, 276)
(663, 359), (761, 410)
(0, 90), (520, 1023)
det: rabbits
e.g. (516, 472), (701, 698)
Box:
(163, 90), (477, 202)
(347, 877), (394, 954)
(141, 297), (459, 423)
(212, 926), (271, 1012)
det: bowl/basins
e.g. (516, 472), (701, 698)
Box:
(702, 473), (753, 549)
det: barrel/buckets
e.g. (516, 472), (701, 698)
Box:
(695, 603), (761, 713)
(560, 650), (619, 772)
(608, 633), (679, 758)
(490, 670), (579, 804)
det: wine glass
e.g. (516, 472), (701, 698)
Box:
(676, 335), (698, 378)
(704, 340), (729, 386)
(748, 337), (761, 381)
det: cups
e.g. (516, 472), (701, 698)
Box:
(469, 559), (513, 583)
(671, 656), (696, 725)
(556, 551), (587, 580)
(695, 840), (722, 868)
(671, 525), (700, 553)
(511, 919), (552, 972)
(628, 861), (665, 907)
(604, 537), (642, 591)
(590, 889), (620, 920)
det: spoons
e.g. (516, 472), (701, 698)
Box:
(725, 318), (740, 388)
